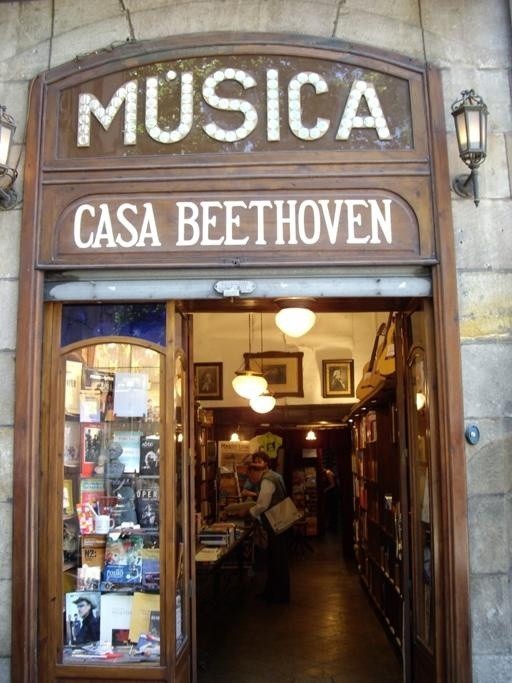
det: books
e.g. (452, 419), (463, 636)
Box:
(61, 358), (161, 665)
(196, 520), (244, 564)
(197, 428), (210, 517)
(348, 407), (378, 592)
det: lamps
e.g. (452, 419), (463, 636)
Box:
(0, 105), (18, 212)
(451, 87), (488, 209)
(232, 297), (317, 415)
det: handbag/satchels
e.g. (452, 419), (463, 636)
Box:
(263, 495), (304, 535)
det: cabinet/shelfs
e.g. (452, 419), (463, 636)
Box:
(193, 378), (405, 664)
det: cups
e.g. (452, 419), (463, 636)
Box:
(94, 513), (115, 535)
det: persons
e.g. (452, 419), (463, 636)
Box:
(245, 451), (294, 606)
(330, 369), (348, 391)
(197, 369), (215, 392)
(240, 463), (260, 500)
(323, 465), (340, 533)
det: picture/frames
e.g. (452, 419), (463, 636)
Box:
(322, 359), (354, 398)
(193, 361), (224, 400)
